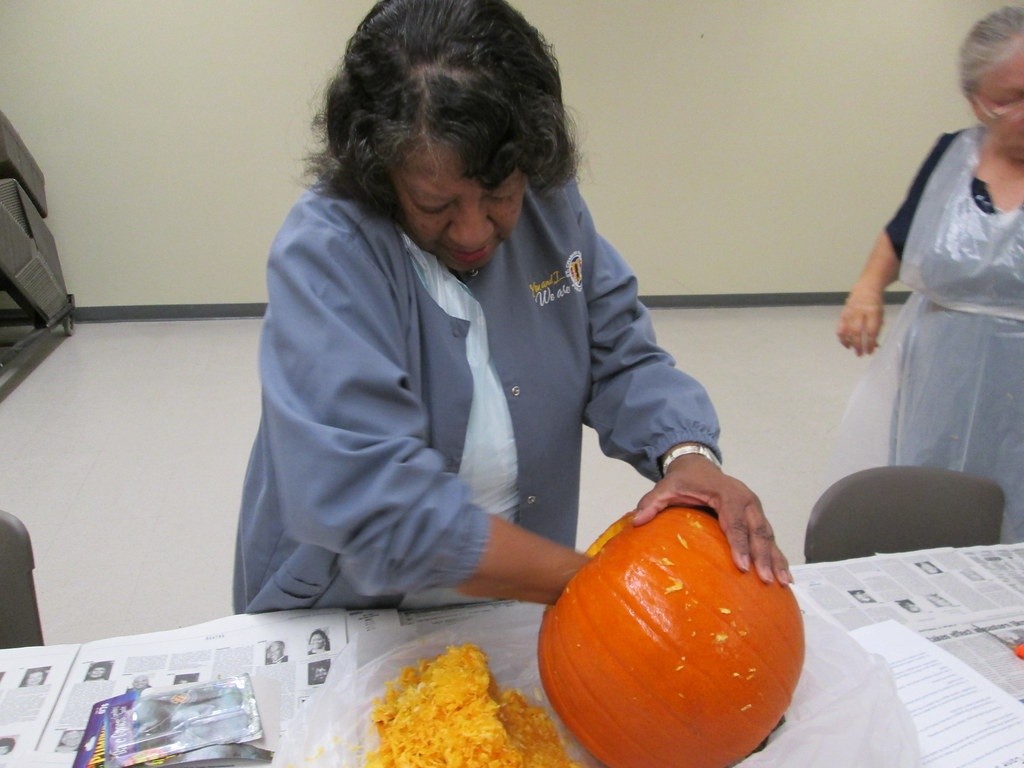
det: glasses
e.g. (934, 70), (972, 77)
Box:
(970, 89), (1024, 124)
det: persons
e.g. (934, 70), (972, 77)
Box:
(87, 662), (109, 680)
(0, 738), (16, 755)
(24, 669), (45, 687)
(308, 629), (330, 654)
(837, 7), (1023, 551)
(227, 2), (794, 616)
(267, 640), (288, 664)
(919, 562), (939, 575)
(309, 662), (328, 686)
(849, 589), (875, 604)
(56, 730), (83, 752)
(899, 598), (921, 613)
(125, 674), (152, 694)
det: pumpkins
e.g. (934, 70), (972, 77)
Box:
(537, 499), (806, 768)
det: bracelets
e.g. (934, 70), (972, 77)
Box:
(661, 444), (727, 476)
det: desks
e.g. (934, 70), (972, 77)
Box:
(2, 544), (1023, 766)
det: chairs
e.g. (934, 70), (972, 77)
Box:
(804, 466), (1004, 567)
(0, 510), (45, 650)
